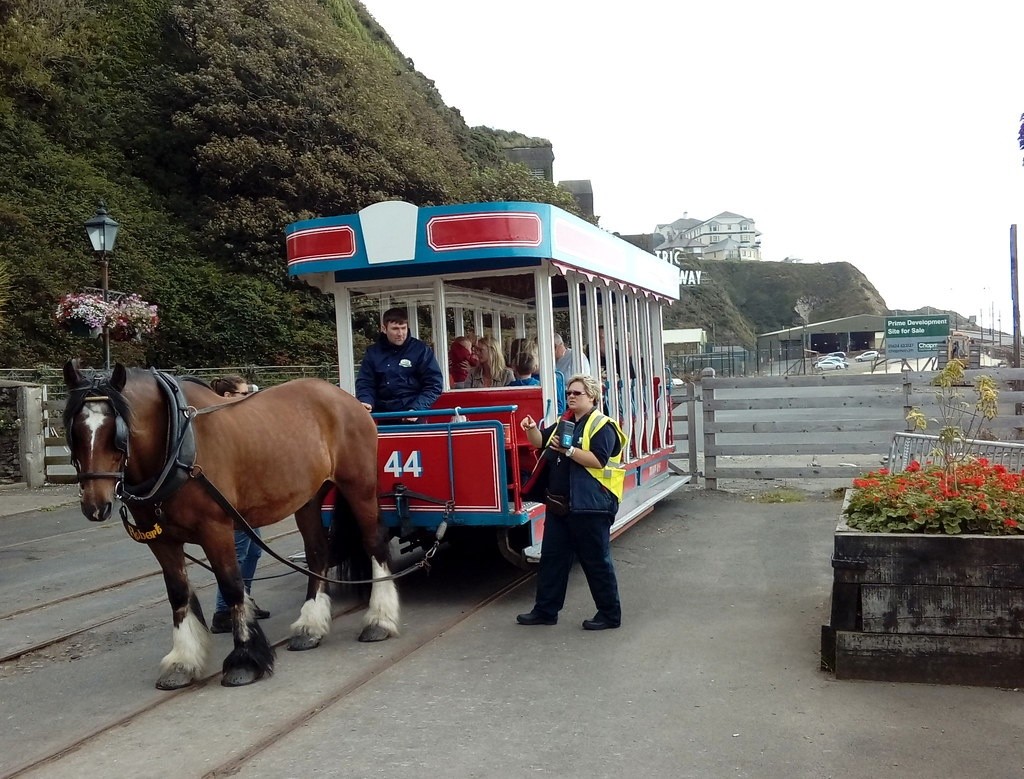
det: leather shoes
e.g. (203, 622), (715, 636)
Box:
(517, 610), (558, 625)
(583, 616), (619, 629)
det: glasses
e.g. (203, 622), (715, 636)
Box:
(230, 390), (249, 396)
(565, 389), (587, 396)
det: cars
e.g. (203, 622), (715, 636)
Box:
(666, 378), (686, 390)
(812, 352), (849, 370)
(855, 351), (880, 362)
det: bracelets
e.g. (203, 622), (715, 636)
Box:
(571, 448), (575, 456)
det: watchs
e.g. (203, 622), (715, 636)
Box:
(565, 446), (574, 457)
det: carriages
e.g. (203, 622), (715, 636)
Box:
(63, 200), (692, 687)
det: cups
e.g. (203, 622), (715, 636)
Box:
(456, 382), (464, 389)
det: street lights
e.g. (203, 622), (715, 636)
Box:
(82, 200), (122, 369)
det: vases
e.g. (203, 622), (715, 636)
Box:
(821, 488), (1024, 684)
(66, 318), (91, 337)
(113, 329), (137, 342)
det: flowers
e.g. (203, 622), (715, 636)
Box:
(841, 356), (1024, 535)
(53, 290), (158, 342)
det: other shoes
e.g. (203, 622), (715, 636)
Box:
(211, 612), (232, 634)
(254, 605), (269, 619)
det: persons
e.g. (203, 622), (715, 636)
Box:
(210, 375), (271, 633)
(356, 307), (444, 426)
(448, 325), (644, 412)
(516, 373), (629, 630)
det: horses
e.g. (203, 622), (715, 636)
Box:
(63, 356), (400, 689)
